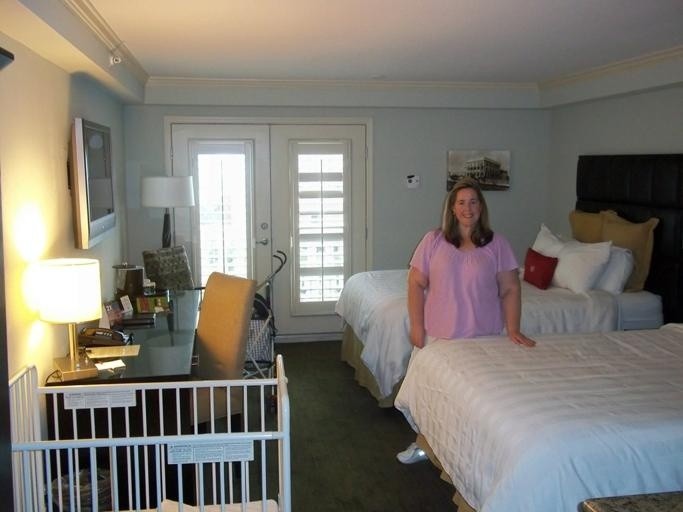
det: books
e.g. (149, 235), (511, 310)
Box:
(118, 312), (156, 325)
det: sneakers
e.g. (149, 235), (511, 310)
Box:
(396, 442), (430, 464)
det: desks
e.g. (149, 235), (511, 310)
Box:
(48, 286), (199, 479)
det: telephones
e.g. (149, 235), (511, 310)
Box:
(77, 328), (132, 347)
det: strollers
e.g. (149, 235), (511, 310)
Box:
(241, 249), (288, 412)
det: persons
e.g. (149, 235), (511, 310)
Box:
(151, 297), (165, 312)
(394, 178), (536, 466)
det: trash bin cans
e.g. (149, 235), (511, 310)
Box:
(52, 468), (111, 512)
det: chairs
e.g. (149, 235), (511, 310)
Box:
(184, 272), (257, 474)
(141, 245), (195, 292)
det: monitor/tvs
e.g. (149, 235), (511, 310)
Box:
(67, 116), (118, 251)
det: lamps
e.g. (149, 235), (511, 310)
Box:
(141, 175), (196, 248)
(37, 256), (102, 382)
(147, 311), (197, 374)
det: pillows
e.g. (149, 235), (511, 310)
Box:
(598, 246), (636, 294)
(602, 212), (661, 290)
(548, 237), (612, 294)
(522, 247), (559, 291)
(568, 207), (615, 243)
(531, 222), (566, 260)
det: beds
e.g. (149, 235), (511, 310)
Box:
(335, 252), (667, 411)
(393, 319), (683, 511)
(6, 352), (294, 511)
